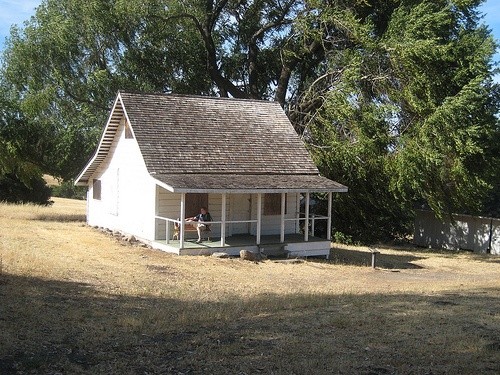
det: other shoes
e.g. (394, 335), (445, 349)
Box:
(196, 240), (202, 243)
(177, 218), (180, 227)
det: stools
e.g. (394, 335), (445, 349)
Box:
(368, 247), (381, 269)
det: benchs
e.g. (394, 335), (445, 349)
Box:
(171, 217), (213, 242)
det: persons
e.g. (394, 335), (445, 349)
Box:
(185, 206), (212, 244)
